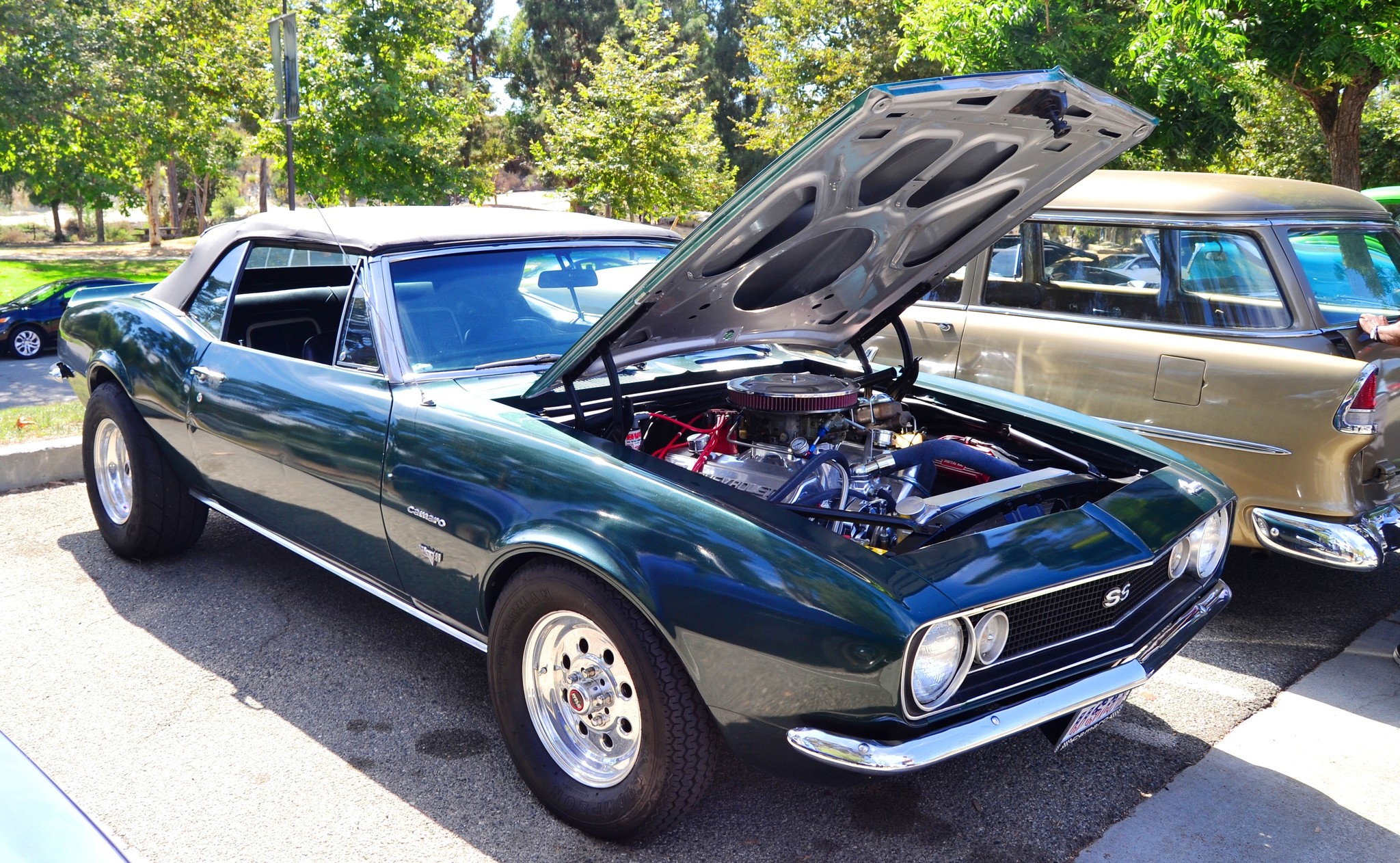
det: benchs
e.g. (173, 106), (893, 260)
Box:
(211, 280), (433, 367)
(132, 234), (179, 242)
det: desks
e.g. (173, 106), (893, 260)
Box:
(134, 227), (181, 235)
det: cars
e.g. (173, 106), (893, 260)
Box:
(842, 162), (1400, 582)
(50, 60), (1243, 851)
(0, 276), (143, 359)
(518, 252), (660, 317)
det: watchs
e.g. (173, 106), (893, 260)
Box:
(1370, 324), (1387, 343)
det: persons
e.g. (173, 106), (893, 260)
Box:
(1359, 313), (1400, 346)
